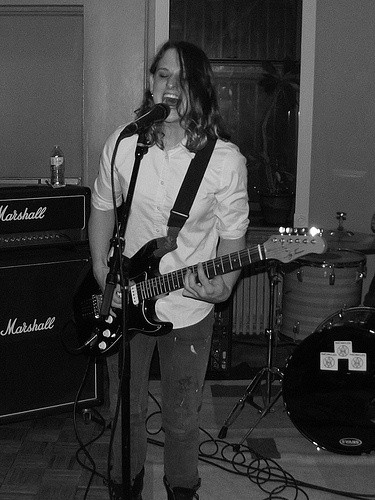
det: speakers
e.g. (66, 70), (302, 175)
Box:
(0, 243), (108, 422)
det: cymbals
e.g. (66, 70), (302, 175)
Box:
(322, 227), (375, 250)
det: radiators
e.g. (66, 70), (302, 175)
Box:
(233, 274), (279, 335)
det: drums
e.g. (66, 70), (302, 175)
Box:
(282, 306), (375, 456)
(277, 248), (367, 344)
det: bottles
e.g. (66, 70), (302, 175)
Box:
(50, 146), (65, 186)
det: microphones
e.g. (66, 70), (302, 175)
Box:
(119, 103), (170, 138)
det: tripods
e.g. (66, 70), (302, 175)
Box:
(216, 265), (286, 438)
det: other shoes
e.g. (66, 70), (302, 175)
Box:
(163, 475), (199, 500)
(112, 466), (144, 500)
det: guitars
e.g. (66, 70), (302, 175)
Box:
(72, 225), (328, 359)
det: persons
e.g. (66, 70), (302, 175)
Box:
(88, 40), (250, 500)
